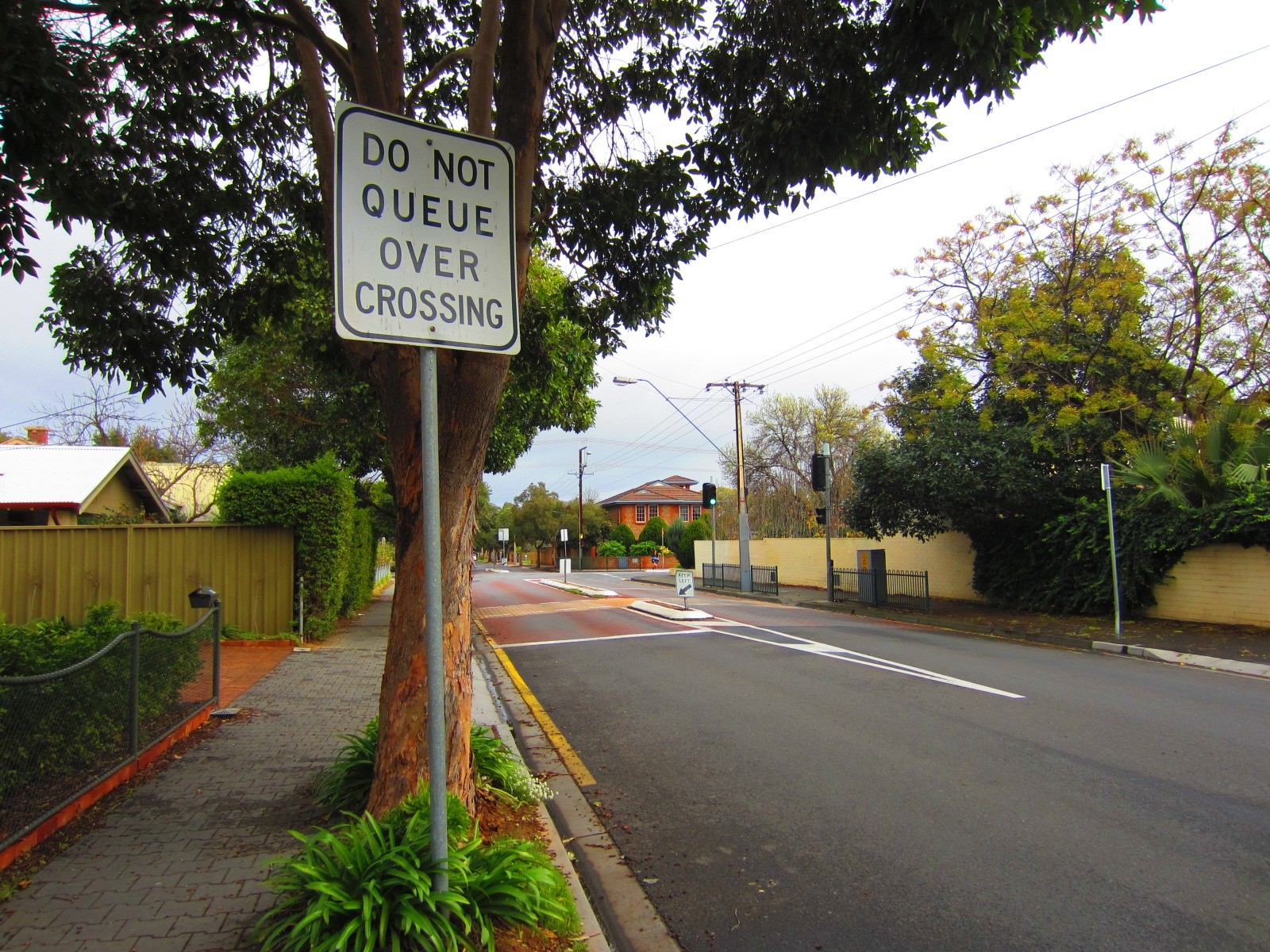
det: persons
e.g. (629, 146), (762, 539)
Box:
(651, 550), (659, 570)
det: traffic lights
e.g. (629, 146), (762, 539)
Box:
(816, 507), (825, 525)
(703, 483), (716, 509)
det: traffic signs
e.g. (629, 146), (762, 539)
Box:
(501, 558), (508, 566)
(676, 571), (695, 597)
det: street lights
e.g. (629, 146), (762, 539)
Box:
(613, 377), (752, 592)
(579, 452), (592, 571)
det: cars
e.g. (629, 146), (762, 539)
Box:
(473, 554), (477, 561)
